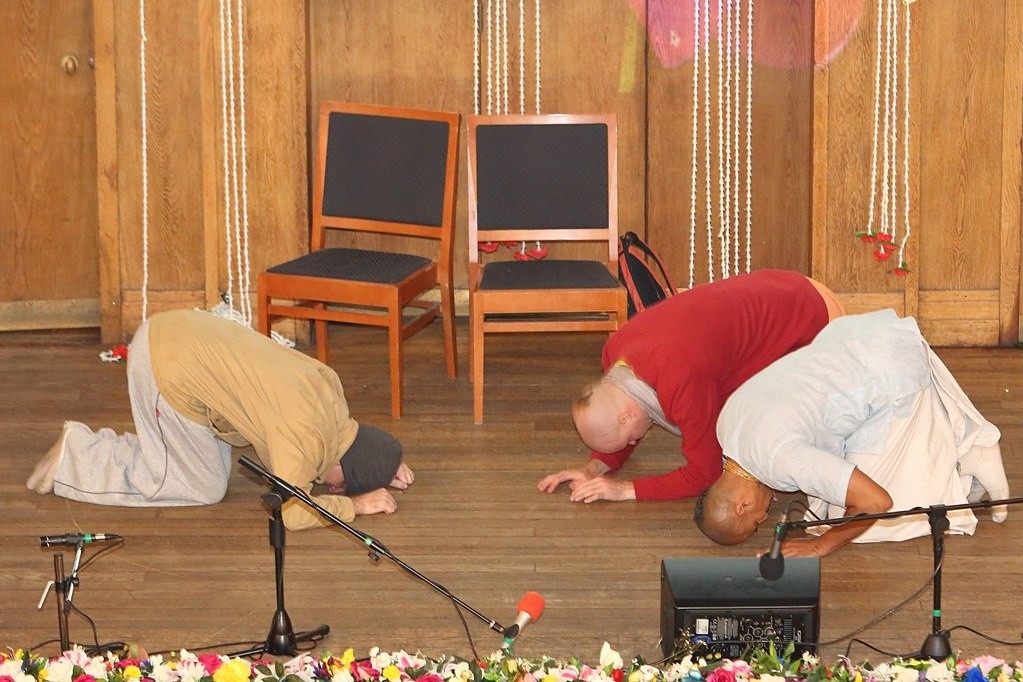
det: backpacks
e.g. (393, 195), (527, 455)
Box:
(616, 229), (678, 317)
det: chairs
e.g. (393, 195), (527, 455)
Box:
(258, 100), (462, 420)
(466, 113), (628, 425)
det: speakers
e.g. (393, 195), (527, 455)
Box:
(660, 556), (821, 665)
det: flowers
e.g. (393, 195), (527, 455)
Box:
(1, 640), (1023, 682)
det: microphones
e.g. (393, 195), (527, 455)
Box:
(759, 505), (790, 580)
(41, 531), (120, 546)
(502, 592), (544, 649)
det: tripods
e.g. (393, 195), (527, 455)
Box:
(225, 455), (505, 661)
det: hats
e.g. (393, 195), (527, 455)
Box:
(340, 423), (403, 495)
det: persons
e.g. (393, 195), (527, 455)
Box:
(26, 309), (415, 532)
(693, 308), (1010, 557)
(538, 269), (847, 504)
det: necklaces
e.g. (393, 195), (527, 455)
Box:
(724, 461), (759, 483)
